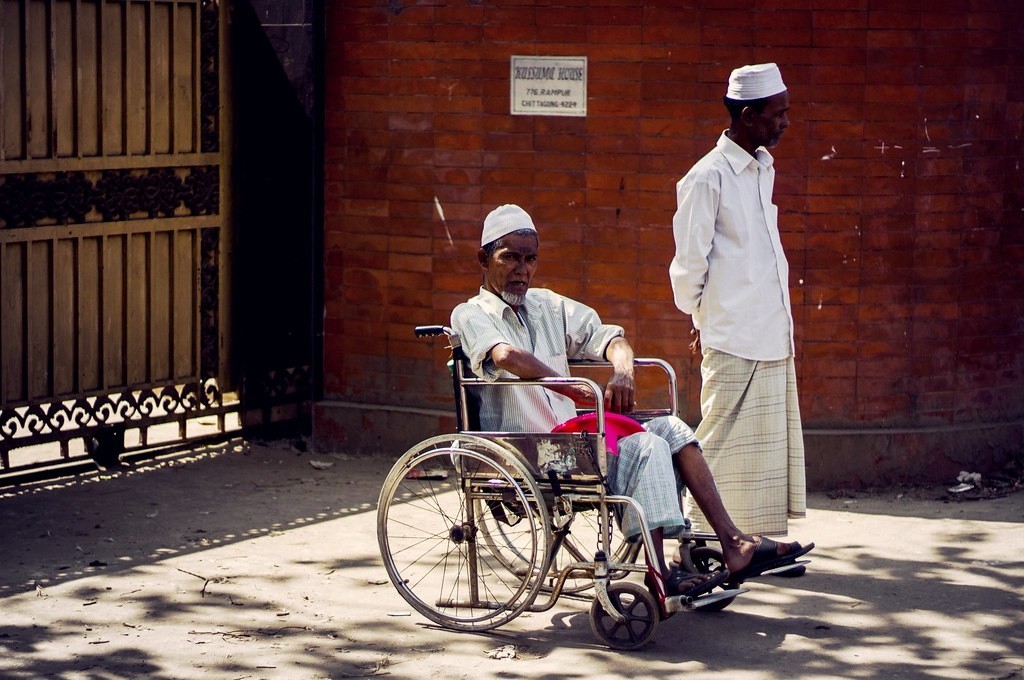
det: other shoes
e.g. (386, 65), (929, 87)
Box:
(763, 560), (807, 577)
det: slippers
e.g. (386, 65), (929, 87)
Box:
(649, 568), (729, 600)
(721, 536), (815, 583)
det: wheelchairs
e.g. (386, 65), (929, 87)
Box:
(376, 324), (811, 651)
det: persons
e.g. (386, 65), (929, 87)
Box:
(669, 63), (808, 578)
(451, 205), (816, 596)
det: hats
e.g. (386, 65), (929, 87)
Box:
(725, 62), (787, 99)
(481, 203), (537, 247)
